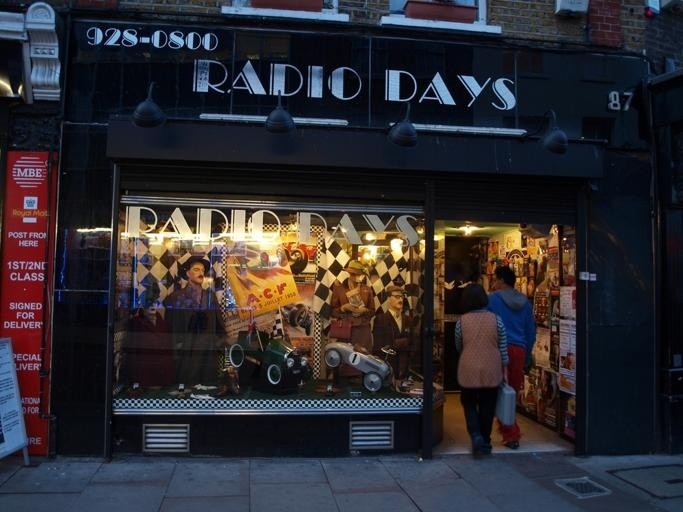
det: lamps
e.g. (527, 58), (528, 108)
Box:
(117, 21), (569, 154)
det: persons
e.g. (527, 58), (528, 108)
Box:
(539, 373), (557, 411)
(455, 265), (537, 464)
(329, 259), (414, 382)
(131, 255), (230, 384)
(564, 353), (575, 369)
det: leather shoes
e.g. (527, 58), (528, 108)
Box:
(471, 434), (492, 459)
(503, 440), (520, 449)
